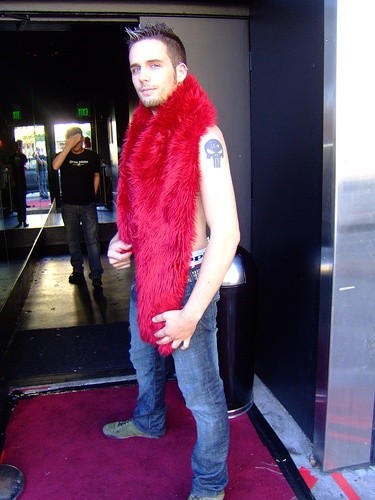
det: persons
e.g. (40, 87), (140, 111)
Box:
(17, 140), (31, 208)
(101, 21), (241, 500)
(84, 137), (92, 151)
(52, 127), (102, 287)
(8, 142), (29, 228)
(32, 148), (50, 201)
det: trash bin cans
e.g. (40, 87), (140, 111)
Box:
(216, 253), (256, 420)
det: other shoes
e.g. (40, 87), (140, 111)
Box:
(69, 271), (84, 280)
(92, 278), (102, 286)
(103, 419), (159, 438)
(188, 489), (225, 500)
